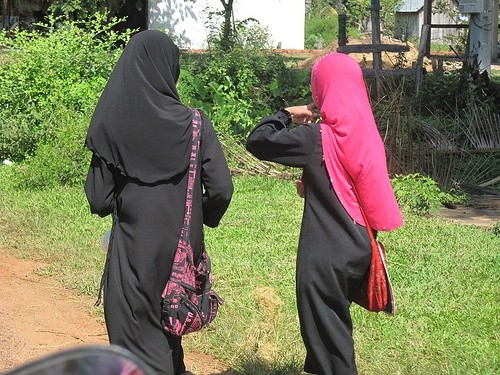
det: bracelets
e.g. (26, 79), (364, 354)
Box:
(281, 108), (292, 126)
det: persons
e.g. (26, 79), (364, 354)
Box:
(246, 53), (403, 375)
(82, 30), (235, 375)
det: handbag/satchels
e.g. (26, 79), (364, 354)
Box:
(160, 242), (224, 336)
(350, 242), (395, 316)
